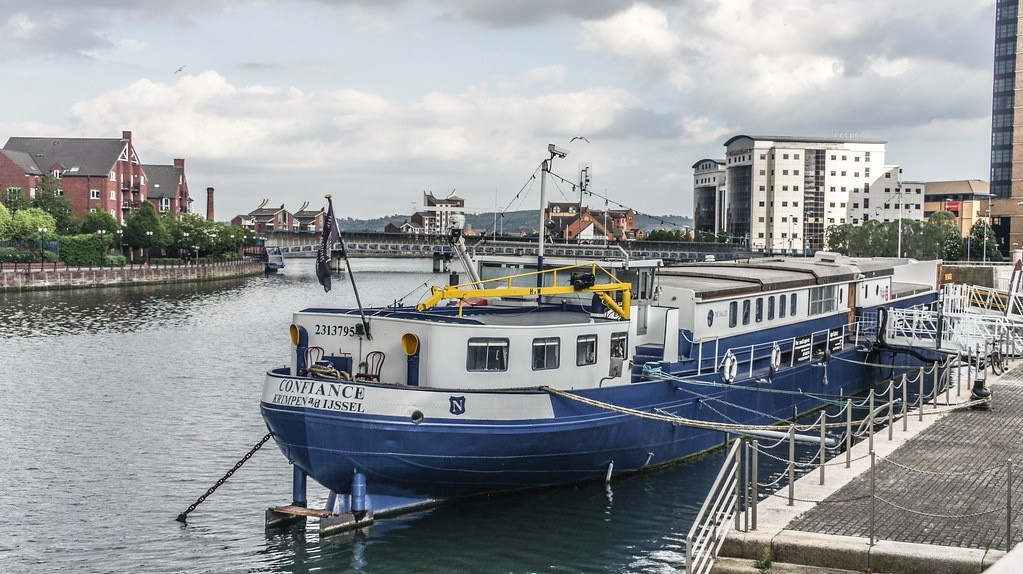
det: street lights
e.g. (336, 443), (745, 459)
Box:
(183, 232), (190, 261)
(116, 230), (122, 255)
(97, 230), (106, 267)
(147, 231), (154, 268)
(212, 234), (216, 264)
(253, 236), (267, 257)
(983, 210), (991, 265)
(231, 234), (234, 262)
(243, 235), (247, 253)
(38, 227), (47, 270)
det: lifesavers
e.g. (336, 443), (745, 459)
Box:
(771, 345), (781, 372)
(724, 354), (737, 383)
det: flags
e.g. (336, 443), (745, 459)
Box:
(316, 205), (345, 293)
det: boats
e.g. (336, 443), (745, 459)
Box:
(261, 158), (944, 533)
(243, 245), (285, 273)
(327, 250), (347, 273)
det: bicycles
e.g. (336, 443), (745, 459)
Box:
(988, 339), (1005, 377)
